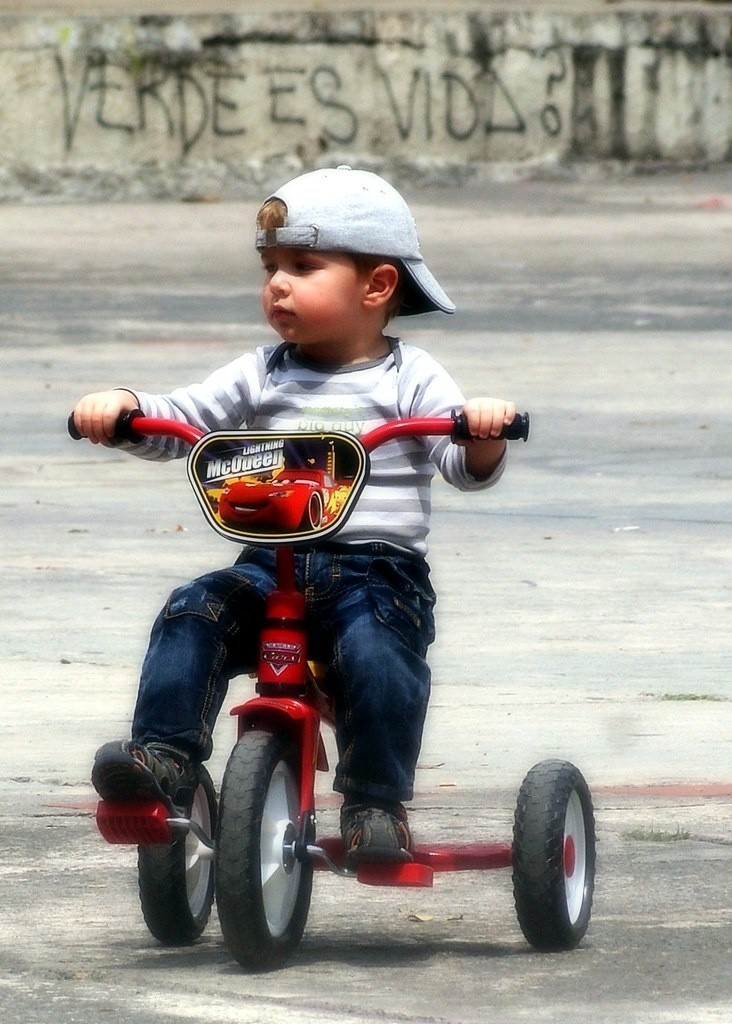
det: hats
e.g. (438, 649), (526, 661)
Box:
(257, 164), (456, 320)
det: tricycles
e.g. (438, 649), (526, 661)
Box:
(69, 407), (597, 971)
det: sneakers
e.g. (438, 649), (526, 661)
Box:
(88, 737), (202, 824)
(340, 795), (414, 867)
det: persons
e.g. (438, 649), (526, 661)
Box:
(72, 165), (516, 867)
